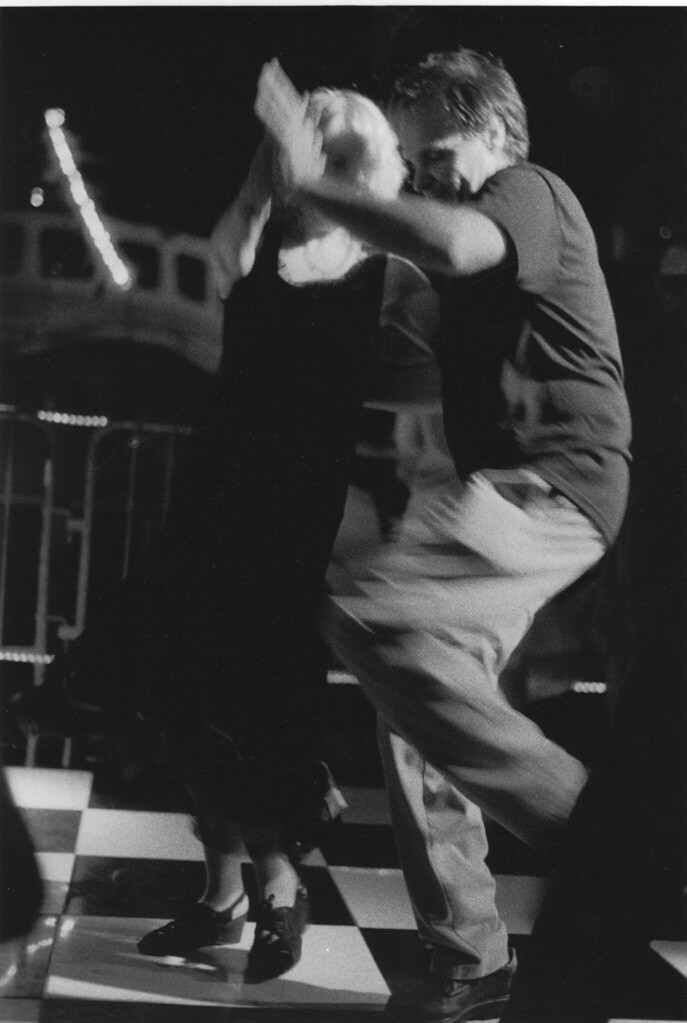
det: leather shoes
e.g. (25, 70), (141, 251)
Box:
(383, 948), (518, 1023)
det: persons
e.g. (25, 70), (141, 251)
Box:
(255, 47), (632, 1022)
(20, 86), (440, 985)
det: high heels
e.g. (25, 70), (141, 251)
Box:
(137, 890), (250, 955)
(243, 877), (309, 983)
